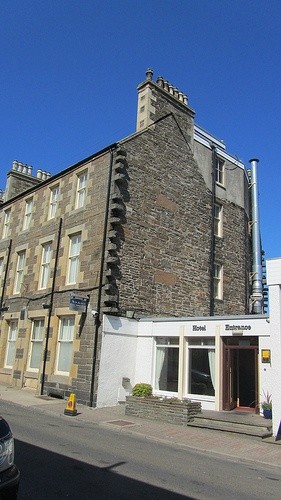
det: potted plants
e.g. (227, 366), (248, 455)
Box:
(248, 390), (272, 419)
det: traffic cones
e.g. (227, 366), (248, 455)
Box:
(64, 394), (77, 416)
